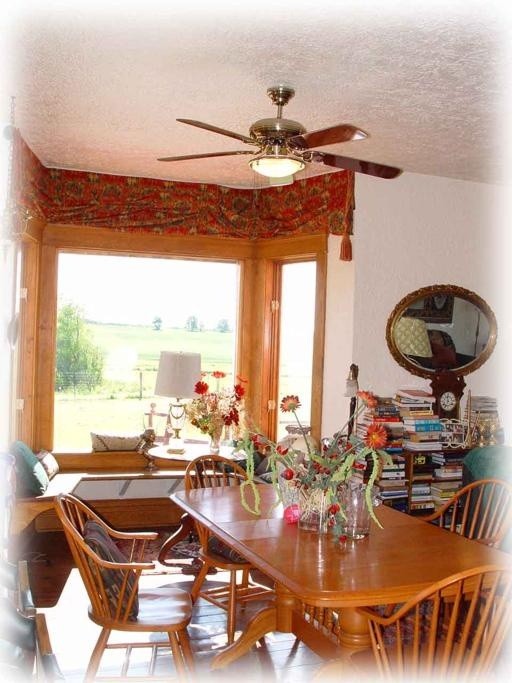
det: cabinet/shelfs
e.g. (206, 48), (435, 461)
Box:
(360, 393), (507, 528)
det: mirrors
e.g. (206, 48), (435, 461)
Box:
(386, 285), (498, 379)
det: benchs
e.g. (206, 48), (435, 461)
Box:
(10, 452), (249, 530)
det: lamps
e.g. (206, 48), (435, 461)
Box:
(155, 349), (202, 454)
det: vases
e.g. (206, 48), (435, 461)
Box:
(208, 428), (221, 450)
(278, 476), (371, 538)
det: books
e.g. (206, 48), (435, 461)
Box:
(356, 452), (408, 514)
(393, 390), (442, 451)
(355, 394), (404, 453)
(411, 453), (469, 511)
(463, 395), (500, 430)
(437, 523), (465, 534)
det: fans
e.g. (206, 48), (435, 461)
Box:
(158, 108), (404, 181)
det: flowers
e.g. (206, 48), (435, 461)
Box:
(253, 392), (384, 541)
(186, 370), (244, 456)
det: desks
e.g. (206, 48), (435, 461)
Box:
(148, 444), (246, 540)
(171, 475), (512, 667)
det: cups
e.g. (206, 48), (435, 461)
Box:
(346, 485), (378, 538)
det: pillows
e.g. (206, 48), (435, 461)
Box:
(8, 442), (49, 496)
(89, 430), (146, 451)
(35, 446), (60, 479)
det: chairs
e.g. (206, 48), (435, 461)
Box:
(49, 493), (195, 683)
(184, 455), (288, 646)
(413, 479), (512, 550)
(345, 563), (512, 683)
